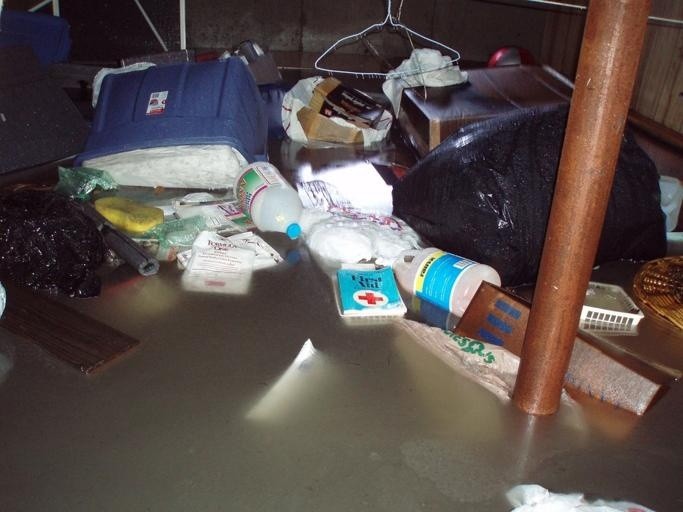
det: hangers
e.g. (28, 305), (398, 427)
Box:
(314, 0), (461, 100)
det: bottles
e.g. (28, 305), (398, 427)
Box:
(391, 247), (503, 319)
(232, 161), (304, 239)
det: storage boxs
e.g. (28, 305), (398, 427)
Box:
(454, 280), (683, 436)
